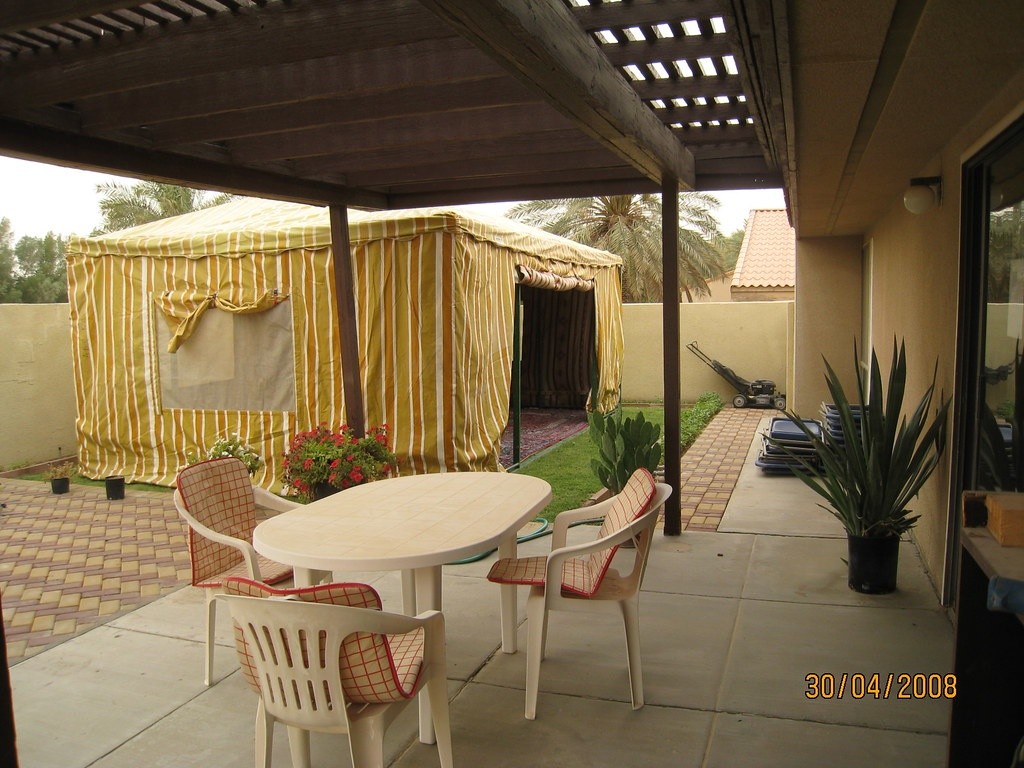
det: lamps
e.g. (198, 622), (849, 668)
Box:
(903, 175), (943, 213)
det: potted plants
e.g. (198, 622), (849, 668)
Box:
(585, 407), (663, 547)
(43, 460), (73, 494)
(759, 331), (953, 596)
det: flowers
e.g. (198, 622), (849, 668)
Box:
(278, 418), (397, 497)
(186, 432), (265, 479)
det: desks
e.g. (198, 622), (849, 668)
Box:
(251, 472), (552, 745)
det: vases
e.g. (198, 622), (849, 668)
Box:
(309, 482), (336, 502)
(106, 475), (125, 500)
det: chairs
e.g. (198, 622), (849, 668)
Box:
(486, 468), (673, 722)
(214, 576), (455, 768)
(172, 457), (333, 687)
(755, 400), (870, 475)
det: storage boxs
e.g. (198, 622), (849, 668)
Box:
(985, 495), (1024, 546)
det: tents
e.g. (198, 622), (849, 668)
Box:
(64, 195), (626, 498)
